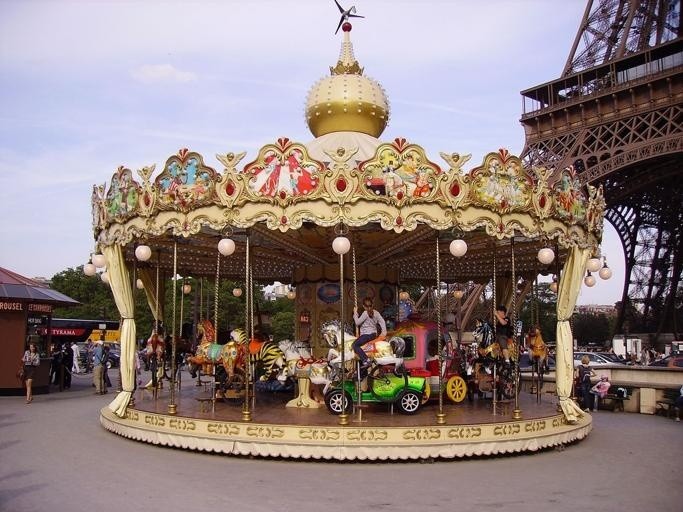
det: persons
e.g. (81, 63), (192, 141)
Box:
(50, 338), (112, 395)
(352, 297), (387, 369)
(674, 385), (683, 422)
(640, 346), (680, 365)
(575, 355), (597, 412)
(589, 374), (611, 412)
(493, 305), (511, 369)
(20, 342), (40, 403)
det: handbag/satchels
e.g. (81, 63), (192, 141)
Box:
(607, 385), (619, 394)
(16, 366), (25, 379)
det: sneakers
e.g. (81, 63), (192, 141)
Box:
(583, 408), (598, 413)
(505, 361), (510, 368)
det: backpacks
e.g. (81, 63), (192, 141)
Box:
(581, 366), (591, 384)
(101, 351), (109, 364)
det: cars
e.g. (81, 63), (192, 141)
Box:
(519, 350), (683, 368)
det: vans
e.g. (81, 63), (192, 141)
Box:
(78, 341), (121, 369)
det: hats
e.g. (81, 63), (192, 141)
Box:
(96, 341), (104, 346)
(600, 374), (608, 379)
